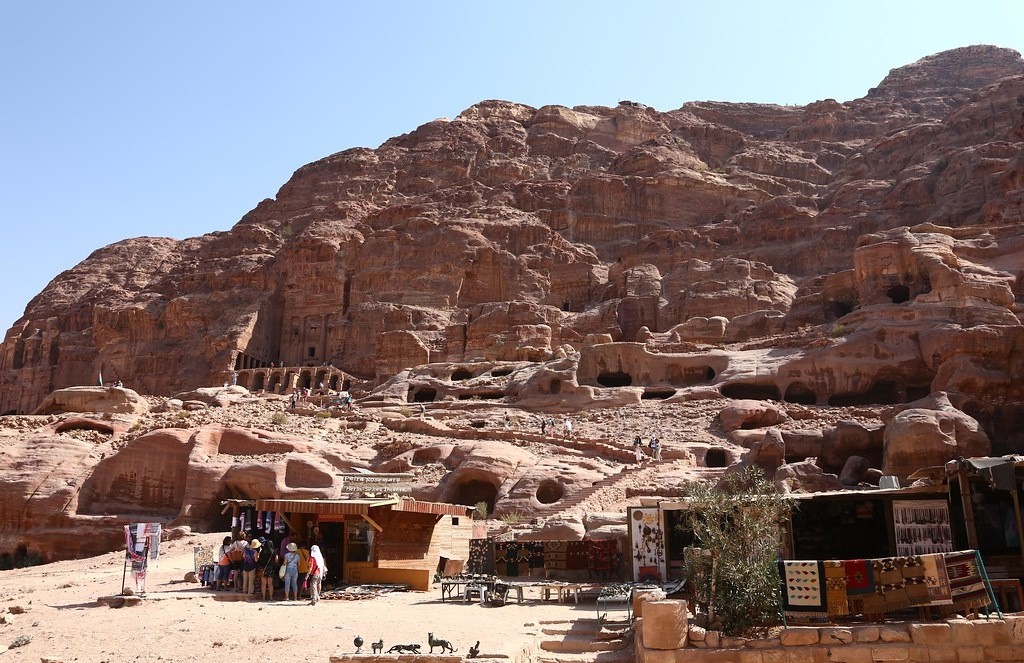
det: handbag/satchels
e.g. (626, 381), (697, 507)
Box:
(307, 574), (313, 588)
(257, 567), (264, 578)
(279, 563), (286, 578)
(218, 552), (230, 566)
(243, 556), (257, 572)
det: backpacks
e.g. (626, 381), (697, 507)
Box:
(233, 540), (246, 561)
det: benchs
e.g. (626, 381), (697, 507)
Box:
(983, 579), (1024, 611)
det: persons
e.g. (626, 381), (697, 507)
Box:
(232, 373), (236, 385)
(503, 412), (510, 431)
(419, 403), (425, 422)
(562, 418), (571, 441)
(648, 434), (661, 461)
(290, 393), (297, 408)
(633, 433), (643, 461)
(215, 531), (327, 605)
(541, 417), (554, 438)
(302, 391), (307, 402)
(346, 394), (352, 411)
(224, 381), (228, 387)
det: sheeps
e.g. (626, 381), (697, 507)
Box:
(372, 640), (383, 654)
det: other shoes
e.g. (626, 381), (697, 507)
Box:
(308, 601), (316, 606)
(279, 597), (290, 601)
(224, 588), (230, 591)
(216, 588), (221, 591)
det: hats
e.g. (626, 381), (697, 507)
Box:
(285, 543), (298, 551)
(258, 536), (267, 542)
(250, 539), (262, 549)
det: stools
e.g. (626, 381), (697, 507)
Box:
(564, 585), (580, 605)
(463, 587), (488, 608)
(540, 586), (564, 604)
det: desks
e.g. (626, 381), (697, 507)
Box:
(596, 586), (633, 641)
(441, 581), (495, 603)
(581, 585), (633, 607)
(517, 584), (550, 603)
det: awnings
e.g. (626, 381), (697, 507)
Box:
(256, 497), (388, 515)
